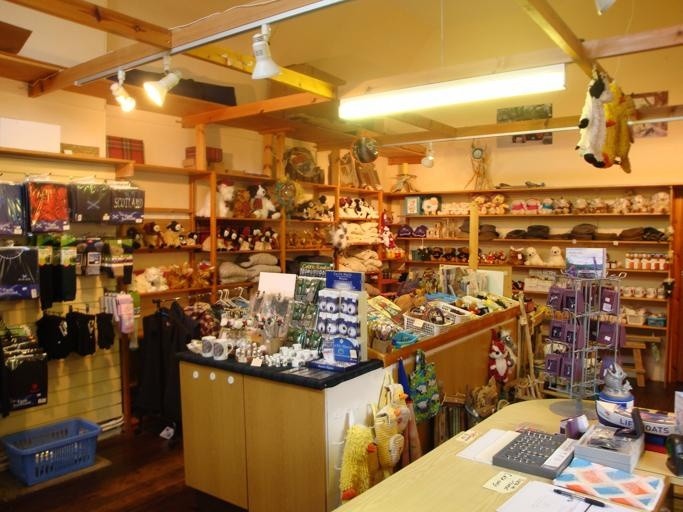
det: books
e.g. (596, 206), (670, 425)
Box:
(493, 423), (666, 512)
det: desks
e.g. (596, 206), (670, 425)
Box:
(329, 398), (683, 512)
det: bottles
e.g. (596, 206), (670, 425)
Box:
(235, 341), (288, 368)
(624, 252), (669, 271)
(606, 257), (617, 268)
(125, 221), (199, 250)
(217, 224), (279, 251)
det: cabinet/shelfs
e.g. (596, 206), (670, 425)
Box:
(179, 360), (384, 512)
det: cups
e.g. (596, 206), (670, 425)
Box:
(202, 336), (217, 357)
(615, 284), (665, 299)
(211, 339), (233, 360)
(279, 343), (319, 368)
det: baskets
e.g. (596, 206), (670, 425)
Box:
(0, 417), (102, 487)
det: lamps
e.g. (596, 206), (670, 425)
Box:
(470, 137), (483, 160)
(421, 142), (435, 168)
(108, 23), (282, 115)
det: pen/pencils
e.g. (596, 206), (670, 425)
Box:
(554, 489), (605, 507)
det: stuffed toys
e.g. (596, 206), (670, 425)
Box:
(575, 69), (615, 169)
(126, 175), (674, 326)
(284, 146), (325, 183)
(602, 81), (635, 173)
(127, 173), (674, 326)
(489, 328), (513, 385)
(338, 373), (411, 504)
(499, 331), (519, 372)
(350, 127), (380, 188)
(261, 146), (281, 174)
